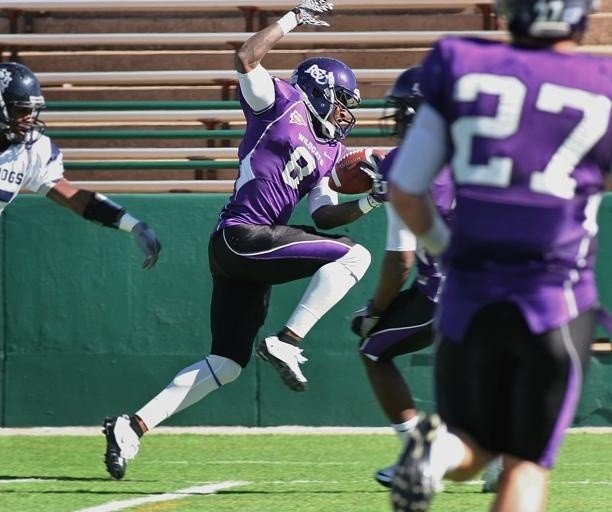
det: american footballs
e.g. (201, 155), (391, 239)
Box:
(328, 149), (388, 194)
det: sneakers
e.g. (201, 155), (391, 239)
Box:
(375, 412), (448, 512)
(102, 414), (140, 480)
(256, 333), (309, 393)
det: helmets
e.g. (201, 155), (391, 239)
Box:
(383, 66), (425, 106)
(291, 57), (361, 124)
(0, 62), (45, 109)
(495, 1), (592, 43)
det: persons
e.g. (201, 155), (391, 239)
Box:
(1, 61), (160, 270)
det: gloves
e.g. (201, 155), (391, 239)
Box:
(131, 221), (161, 270)
(292, 0), (334, 27)
